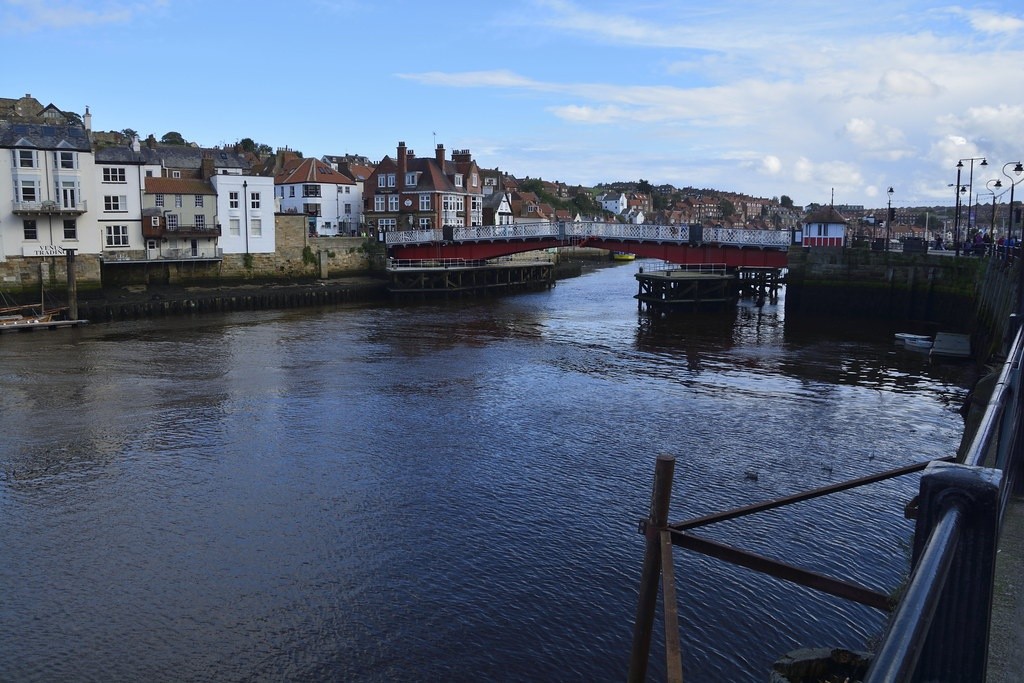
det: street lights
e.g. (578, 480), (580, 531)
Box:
(987, 178), (1002, 244)
(886, 186), (894, 251)
(952, 185), (968, 249)
(957, 157), (989, 241)
(1003, 161), (1024, 246)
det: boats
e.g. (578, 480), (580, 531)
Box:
(0, 282), (69, 330)
(614, 251), (635, 260)
